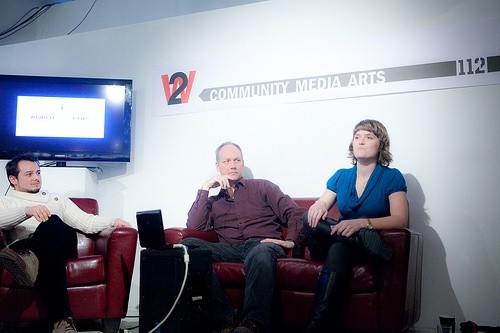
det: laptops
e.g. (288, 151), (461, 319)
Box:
(136, 210), (199, 251)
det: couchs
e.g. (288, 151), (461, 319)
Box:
(164, 198), (424, 333)
(0, 197), (138, 333)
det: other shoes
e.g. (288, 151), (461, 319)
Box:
(233, 320), (260, 333)
(220, 317), (235, 333)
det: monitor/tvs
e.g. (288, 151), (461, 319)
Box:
(0, 75), (133, 168)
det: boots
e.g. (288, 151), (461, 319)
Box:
(303, 270), (340, 333)
(308, 217), (394, 261)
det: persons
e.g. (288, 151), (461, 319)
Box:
(302, 120), (410, 333)
(179, 142), (305, 333)
(0, 155), (130, 333)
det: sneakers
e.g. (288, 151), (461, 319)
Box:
(52, 317), (77, 333)
(0, 248), (38, 287)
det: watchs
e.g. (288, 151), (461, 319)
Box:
(366, 217), (374, 230)
